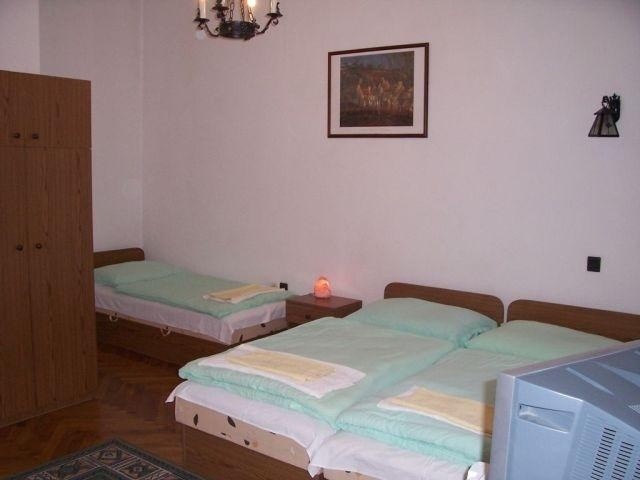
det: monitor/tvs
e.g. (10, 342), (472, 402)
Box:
(489, 340), (640, 480)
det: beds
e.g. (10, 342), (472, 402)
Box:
(306, 299), (640, 480)
(166, 281), (504, 479)
(94, 247), (295, 367)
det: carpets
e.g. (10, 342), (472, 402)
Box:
(6, 436), (205, 480)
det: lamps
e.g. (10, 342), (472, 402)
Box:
(194, 0), (283, 41)
(587, 94), (620, 138)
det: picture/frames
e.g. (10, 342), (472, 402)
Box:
(327, 41), (429, 138)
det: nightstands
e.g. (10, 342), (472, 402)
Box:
(285, 292), (364, 325)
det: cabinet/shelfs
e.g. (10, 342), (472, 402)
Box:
(0, 71), (102, 479)
